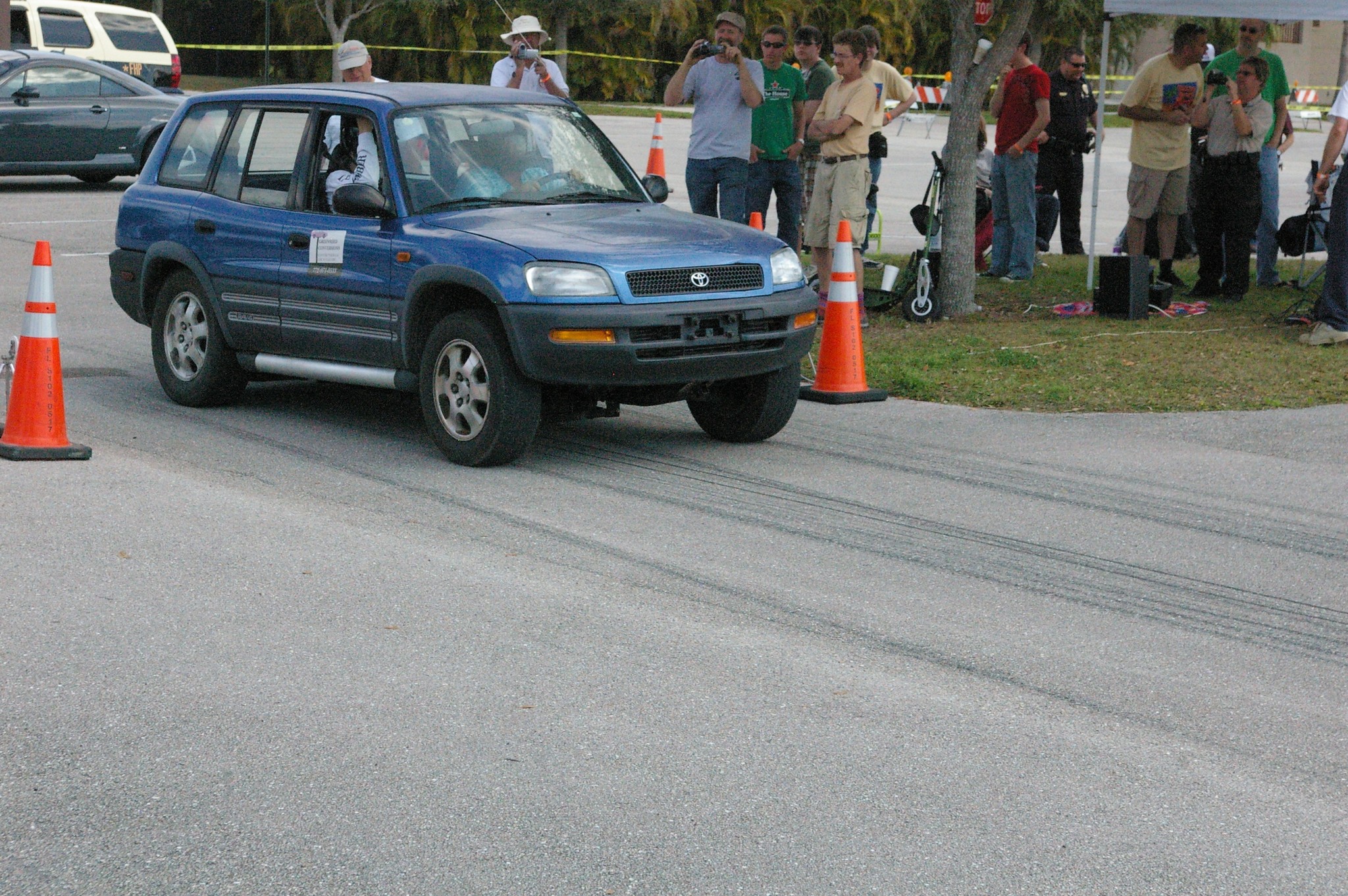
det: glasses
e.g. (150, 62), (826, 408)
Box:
(1065, 58), (1086, 68)
(830, 52), (856, 59)
(763, 41), (785, 48)
(795, 39), (820, 47)
(1235, 68), (1260, 78)
(1239, 24), (1264, 33)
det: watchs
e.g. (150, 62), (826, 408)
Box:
(796, 138), (804, 146)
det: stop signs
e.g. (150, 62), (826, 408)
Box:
(973, 0), (995, 27)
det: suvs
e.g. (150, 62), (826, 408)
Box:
(9, 0), (183, 93)
(103, 80), (824, 467)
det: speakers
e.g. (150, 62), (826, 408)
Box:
(1099, 256), (1149, 320)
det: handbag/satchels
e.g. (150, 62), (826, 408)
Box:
(909, 204), (941, 236)
(1277, 206), (1332, 256)
(868, 131), (888, 157)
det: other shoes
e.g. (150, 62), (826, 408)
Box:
(976, 271), (999, 277)
(1222, 291), (1242, 304)
(1273, 280), (1293, 291)
(860, 309), (869, 326)
(1181, 288), (1217, 299)
(1158, 271), (1189, 289)
(1000, 272), (1027, 283)
(862, 257), (884, 270)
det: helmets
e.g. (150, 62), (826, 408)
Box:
(476, 111), (535, 168)
(341, 115), (377, 151)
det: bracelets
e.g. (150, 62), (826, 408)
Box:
(886, 112), (892, 122)
(1014, 144), (1022, 155)
(1229, 100), (1242, 105)
(1317, 173), (1330, 179)
(542, 73), (551, 82)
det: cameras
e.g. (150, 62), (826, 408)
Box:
(1206, 70), (1227, 85)
(697, 41), (724, 54)
(518, 44), (538, 59)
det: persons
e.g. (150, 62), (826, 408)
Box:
(1035, 44), (1105, 255)
(976, 28), (1051, 283)
(489, 16), (570, 100)
(325, 105), (585, 220)
(941, 113), (994, 226)
(663, 12), (919, 328)
(319, 40), (431, 175)
(1117, 20), (1348, 346)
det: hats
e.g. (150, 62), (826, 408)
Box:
(498, 14), (549, 46)
(713, 12), (746, 32)
(337, 39), (370, 70)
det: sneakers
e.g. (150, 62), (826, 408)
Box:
(1298, 323), (1348, 345)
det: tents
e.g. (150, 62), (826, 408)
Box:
(1086, 0), (1348, 293)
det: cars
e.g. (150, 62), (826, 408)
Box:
(0, 47), (220, 187)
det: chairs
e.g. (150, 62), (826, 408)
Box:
(313, 144), (348, 212)
(442, 140), (478, 205)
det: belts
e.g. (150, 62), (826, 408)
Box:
(823, 153), (870, 164)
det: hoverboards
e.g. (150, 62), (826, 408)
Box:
(810, 150), (988, 324)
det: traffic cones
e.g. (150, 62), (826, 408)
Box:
(797, 219), (891, 404)
(0, 237), (93, 462)
(640, 111), (675, 193)
